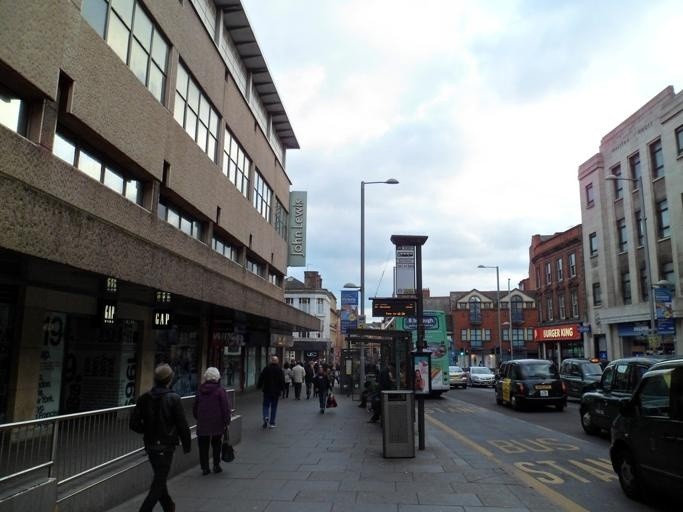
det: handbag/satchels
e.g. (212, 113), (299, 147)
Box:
(221, 427), (234, 463)
(325, 392), (337, 408)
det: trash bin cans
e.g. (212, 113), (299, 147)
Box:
(381, 390), (416, 459)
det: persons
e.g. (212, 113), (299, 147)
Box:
(257, 355), (286, 428)
(192, 366), (232, 475)
(415, 369), (425, 392)
(128, 364), (192, 512)
(283, 358), (394, 423)
(479, 361), (482, 366)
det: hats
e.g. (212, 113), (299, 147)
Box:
(152, 362), (171, 380)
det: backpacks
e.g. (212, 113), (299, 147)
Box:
(127, 389), (177, 434)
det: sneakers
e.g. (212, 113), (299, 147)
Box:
(267, 423), (277, 428)
(262, 416), (269, 428)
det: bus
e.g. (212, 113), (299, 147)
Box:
(378, 306), (452, 402)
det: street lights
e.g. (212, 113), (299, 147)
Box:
(343, 179), (400, 405)
(498, 320), (513, 360)
(477, 264), (504, 363)
(598, 172), (677, 355)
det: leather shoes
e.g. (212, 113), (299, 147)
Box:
(201, 465), (223, 475)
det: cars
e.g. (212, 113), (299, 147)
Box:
(495, 355), (683, 506)
(449, 365), (467, 389)
(465, 367), (496, 387)
